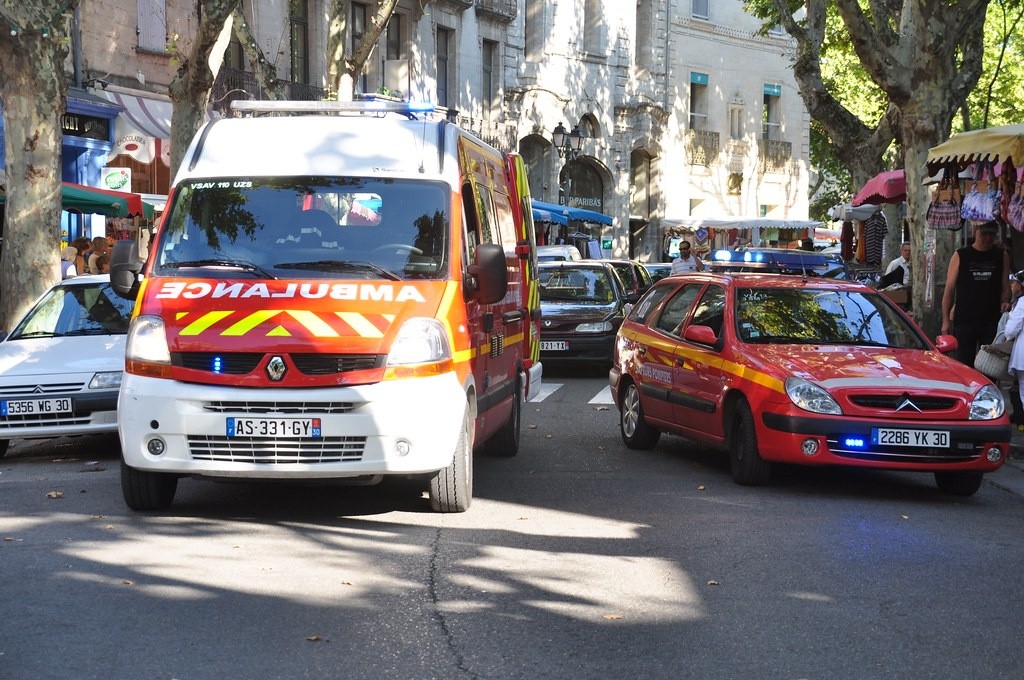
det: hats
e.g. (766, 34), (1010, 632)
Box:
(1008, 270), (1024, 287)
(975, 220), (998, 233)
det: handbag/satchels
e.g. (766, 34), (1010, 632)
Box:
(1007, 167), (1024, 232)
(961, 162), (1000, 222)
(927, 164), (962, 230)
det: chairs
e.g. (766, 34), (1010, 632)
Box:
(214, 201), (429, 252)
(84, 290), (124, 324)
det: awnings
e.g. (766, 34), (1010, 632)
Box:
(629, 214), (824, 236)
(61, 175), (155, 222)
(348, 199), (382, 225)
(531, 197), (613, 228)
(104, 86), (222, 168)
(832, 124), (1024, 221)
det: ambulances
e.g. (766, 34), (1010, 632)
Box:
(118, 102), (542, 520)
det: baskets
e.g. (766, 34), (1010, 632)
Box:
(974, 330), (1009, 379)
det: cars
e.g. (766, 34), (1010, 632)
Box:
(609, 247), (1010, 498)
(1, 274), (143, 455)
(537, 260), (673, 373)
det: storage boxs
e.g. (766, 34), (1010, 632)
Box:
(881, 290), (908, 309)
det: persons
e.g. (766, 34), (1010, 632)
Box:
(62, 236), (111, 276)
(669, 240), (706, 277)
(797, 237), (813, 249)
(884, 242), (912, 290)
(139, 233), (157, 274)
(942, 220), (1024, 431)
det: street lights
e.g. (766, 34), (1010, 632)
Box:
(552, 123), (585, 245)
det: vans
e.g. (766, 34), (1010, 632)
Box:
(537, 245), (584, 261)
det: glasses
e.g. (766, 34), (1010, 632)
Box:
(679, 248), (688, 250)
